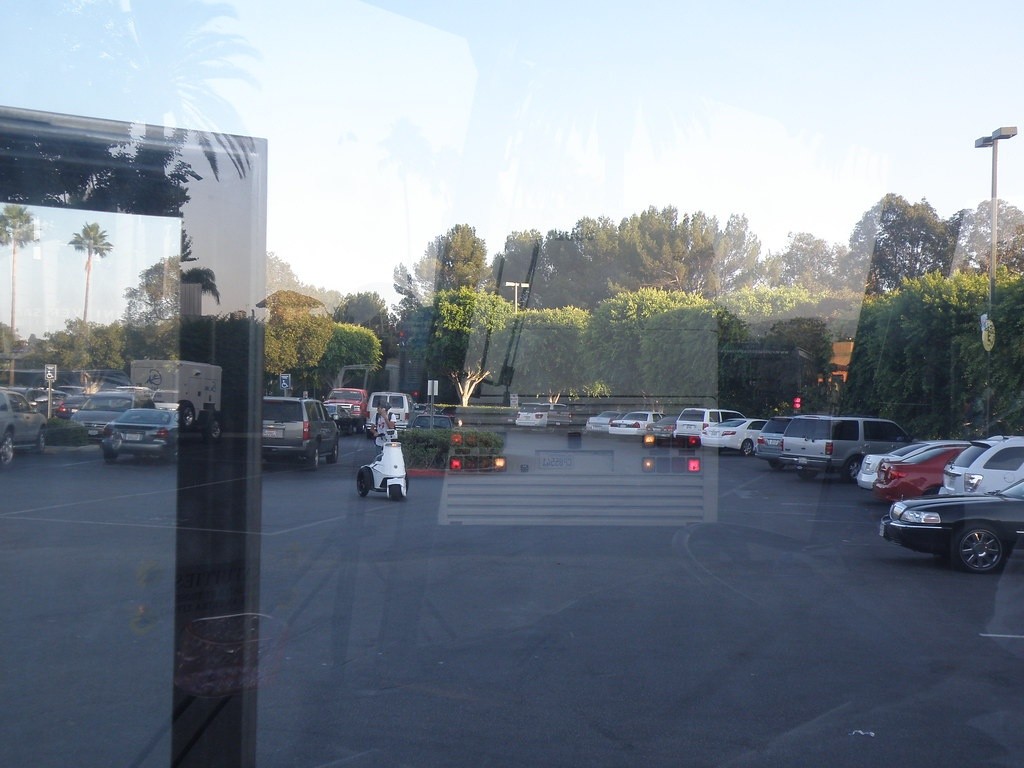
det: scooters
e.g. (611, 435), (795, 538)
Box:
(355, 427), (410, 503)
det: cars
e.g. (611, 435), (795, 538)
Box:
(0, 360), (455, 442)
(855, 440), (979, 503)
(876, 476), (1023, 577)
(939, 433), (1023, 505)
(514, 402), (771, 456)
(755, 417), (794, 471)
(98, 408), (178, 467)
(779, 415), (922, 480)
(261, 398), (341, 469)
(0, 391), (49, 465)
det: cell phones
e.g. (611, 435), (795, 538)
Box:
(382, 408), (385, 410)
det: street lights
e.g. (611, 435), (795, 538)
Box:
(973, 124), (1018, 441)
(504, 280), (531, 316)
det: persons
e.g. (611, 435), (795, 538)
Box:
(368, 399), (398, 460)
